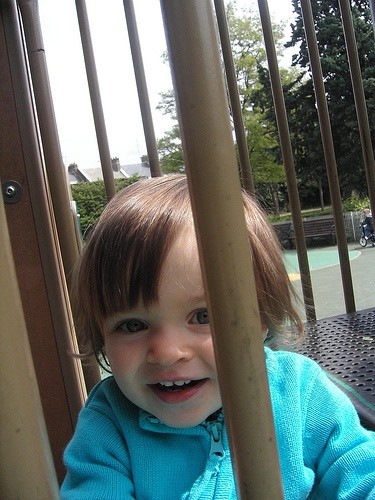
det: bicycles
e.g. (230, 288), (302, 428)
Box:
(358, 220), (375, 248)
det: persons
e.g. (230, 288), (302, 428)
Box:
(59, 173), (375, 500)
(361, 208), (375, 239)
